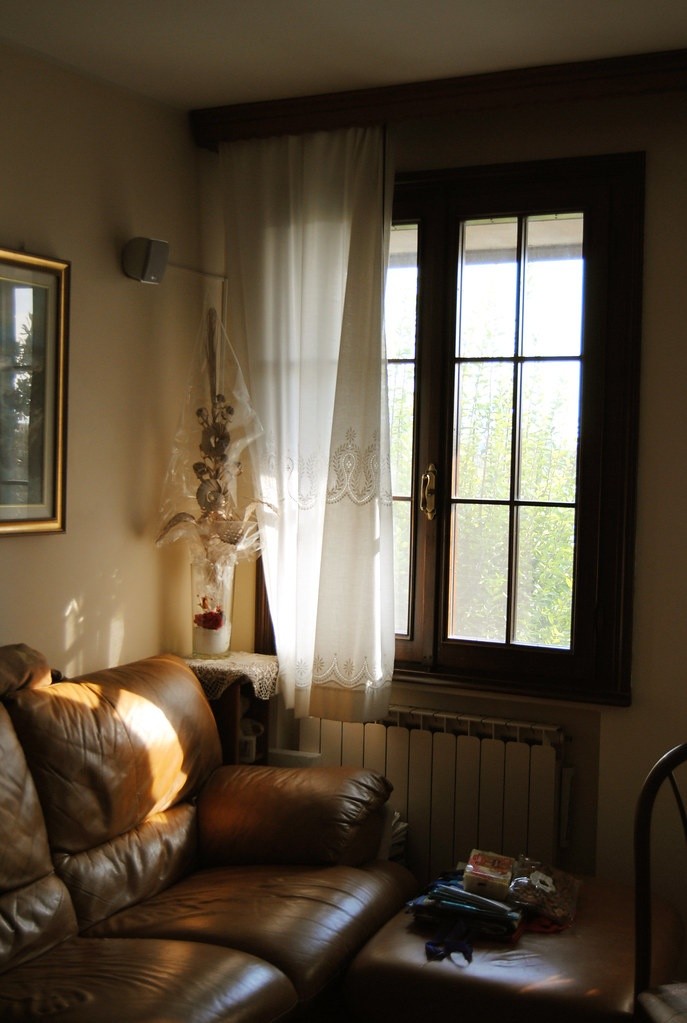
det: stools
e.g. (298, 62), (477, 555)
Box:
(344, 864), (674, 1023)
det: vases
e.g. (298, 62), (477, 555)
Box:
(189, 563), (236, 658)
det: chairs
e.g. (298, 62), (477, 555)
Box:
(631, 739), (687, 1023)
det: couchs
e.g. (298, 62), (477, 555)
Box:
(0, 641), (416, 1023)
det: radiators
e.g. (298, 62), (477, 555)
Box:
(296, 703), (565, 887)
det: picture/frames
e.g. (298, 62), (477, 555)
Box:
(0, 246), (73, 537)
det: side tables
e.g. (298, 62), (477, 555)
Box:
(178, 652), (280, 767)
